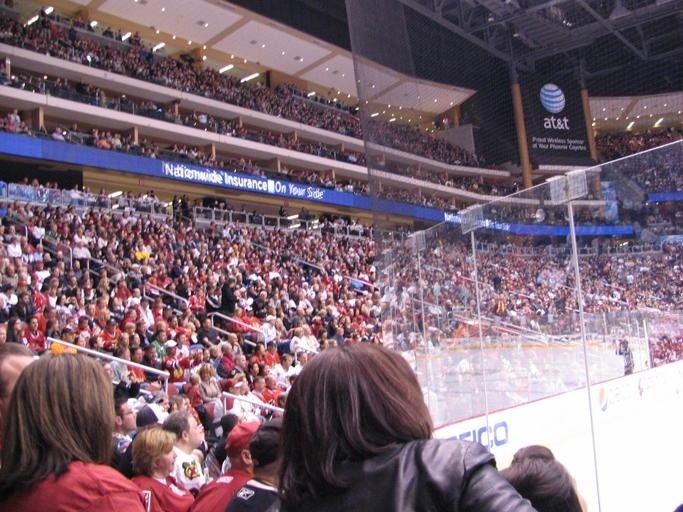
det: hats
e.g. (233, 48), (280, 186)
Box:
(165, 339), (178, 352)
(136, 403), (171, 426)
(225, 420), (262, 456)
(249, 415), (286, 467)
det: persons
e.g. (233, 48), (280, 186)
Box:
(1, 6), (683, 512)
